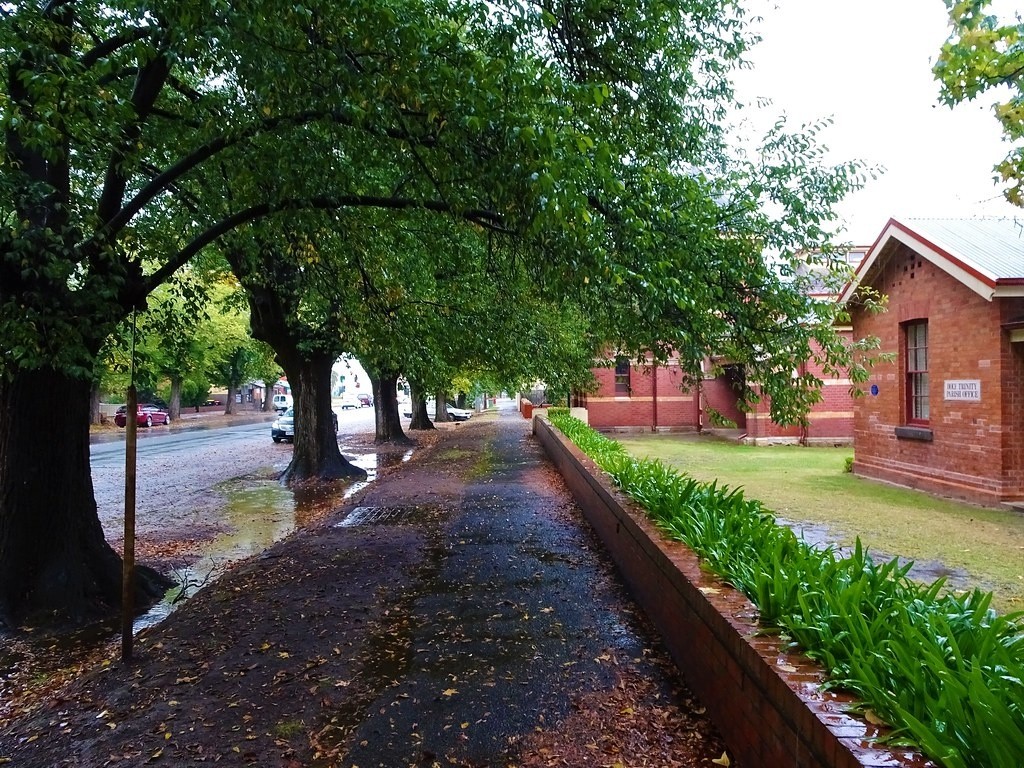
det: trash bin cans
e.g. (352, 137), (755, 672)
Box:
(202, 401), (207, 406)
(214, 401), (220, 405)
(101, 411), (107, 420)
(207, 400), (214, 406)
(99, 411), (102, 419)
(489, 400), (493, 406)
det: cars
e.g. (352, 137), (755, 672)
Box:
(114, 403), (171, 428)
(356, 393), (374, 408)
(403, 400), (474, 422)
(342, 394), (362, 410)
(271, 405), (339, 443)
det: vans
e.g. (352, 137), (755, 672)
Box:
(272, 394), (293, 412)
